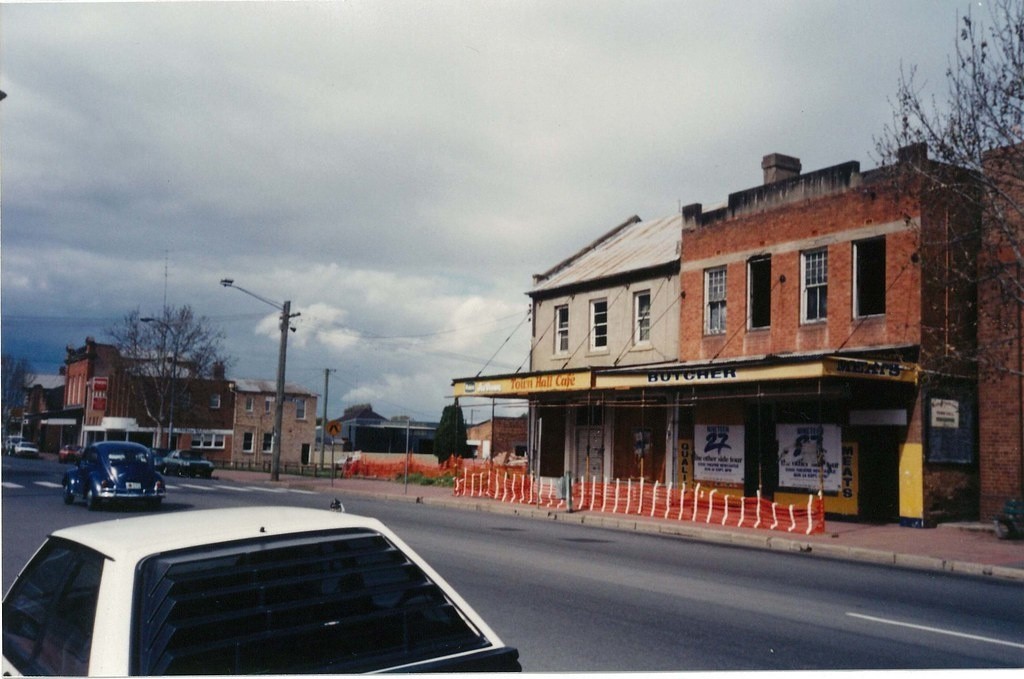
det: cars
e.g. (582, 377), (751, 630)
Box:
(2, 505), (525, 678)
(4, 435), (215, 512)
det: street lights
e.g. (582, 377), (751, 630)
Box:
(140, 317), (178, 451)
(220, 279), (291, 483)
